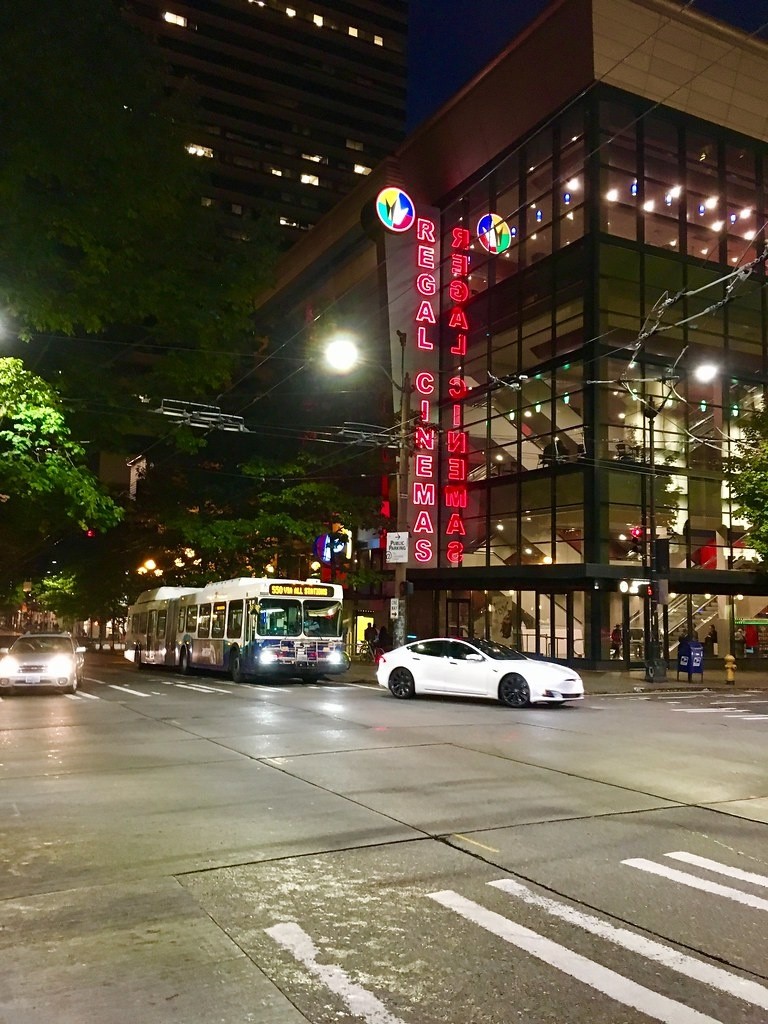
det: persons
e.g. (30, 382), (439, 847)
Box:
(364, 622), (390, 650)
(302, 613), (319, 636)
(611, 623), (748, 659)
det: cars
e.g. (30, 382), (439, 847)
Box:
(0, 630), (88, 697)
(0, 628), (23, 660)
(376, 636), (584, 707)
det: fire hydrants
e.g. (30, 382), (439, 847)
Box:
(723, 653), (737, 685)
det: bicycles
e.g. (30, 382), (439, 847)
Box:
(343, 643), (352, 671)
(359, 640), (385, 665)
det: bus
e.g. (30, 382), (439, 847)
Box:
(124, 576), (345, 685)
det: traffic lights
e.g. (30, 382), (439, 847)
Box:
(632, 527), (646, 555)
(642, 585), (655, 598)
